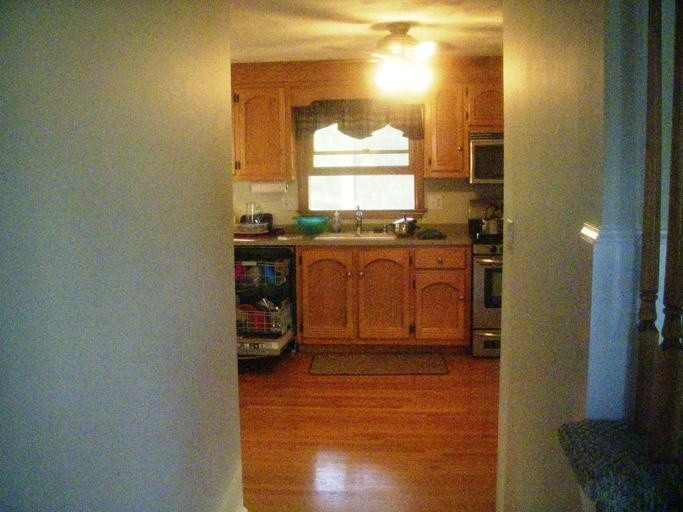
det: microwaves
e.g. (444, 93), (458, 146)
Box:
(470, 138), (504, 183)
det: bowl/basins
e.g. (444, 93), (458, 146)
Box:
(294, 215), (328, 229)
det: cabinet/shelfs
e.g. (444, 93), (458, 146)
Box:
(231, 62), (298, 181)
(423, 55), (469, 180)
(295, 245), (413, 353)
(464, 56), (504, 133)
(409, 245), (472, 354)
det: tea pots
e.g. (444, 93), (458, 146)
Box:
(480, 204), (502, 234)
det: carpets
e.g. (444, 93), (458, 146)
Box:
(309, 352), (450, 376)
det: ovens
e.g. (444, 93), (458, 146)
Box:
(474, 256), (501, 329)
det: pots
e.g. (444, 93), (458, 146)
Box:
(389, 214), (420, 236)
